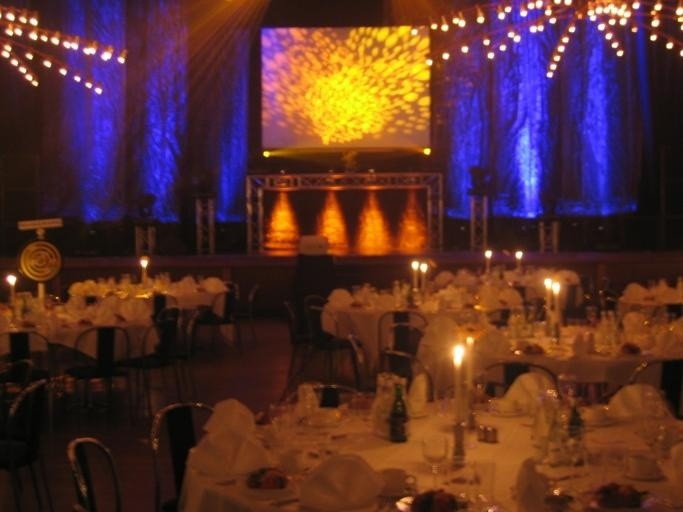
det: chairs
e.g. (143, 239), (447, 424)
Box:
(0, 260), (682, 512)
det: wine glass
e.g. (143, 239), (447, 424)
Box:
(0, 291), (97, 334)
(462, 306), (683, 354)
